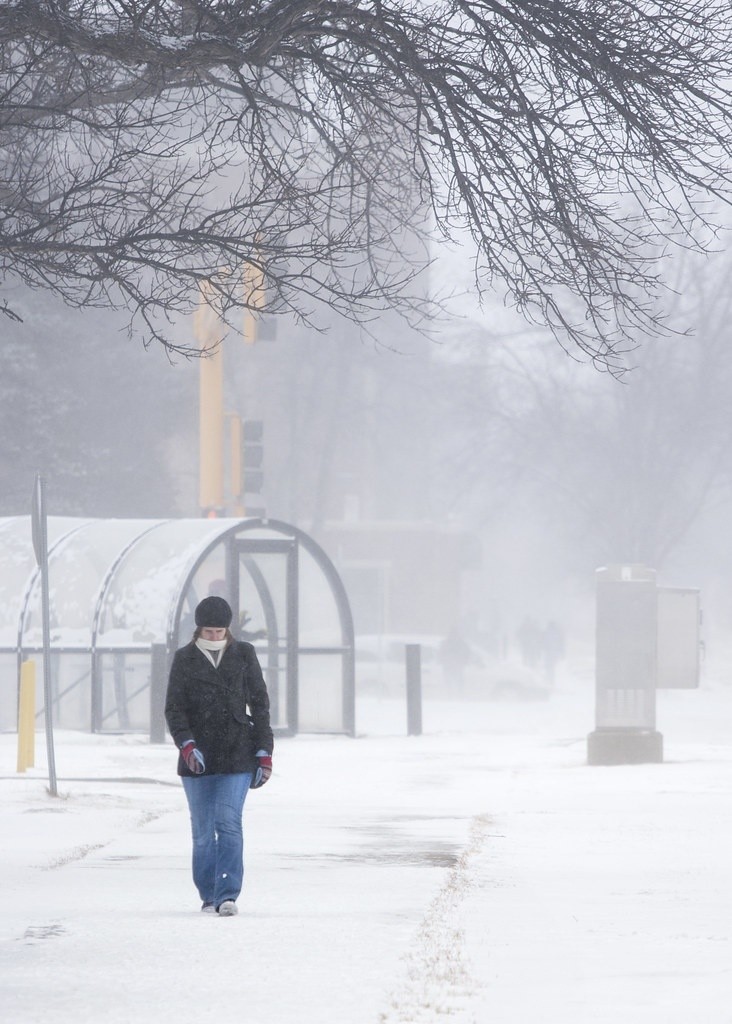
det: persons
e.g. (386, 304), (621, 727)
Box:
(165, 596), (274, 917)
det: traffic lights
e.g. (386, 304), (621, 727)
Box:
(231, 415), (266, 494)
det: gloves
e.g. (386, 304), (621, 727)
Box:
(179, 739), (206, 775)
(249, 749), (273, 789)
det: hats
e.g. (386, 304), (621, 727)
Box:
(194, 596), (233, 628)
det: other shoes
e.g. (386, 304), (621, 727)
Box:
(218, 900), (238, 916)
(200, 902), (216, 917)
